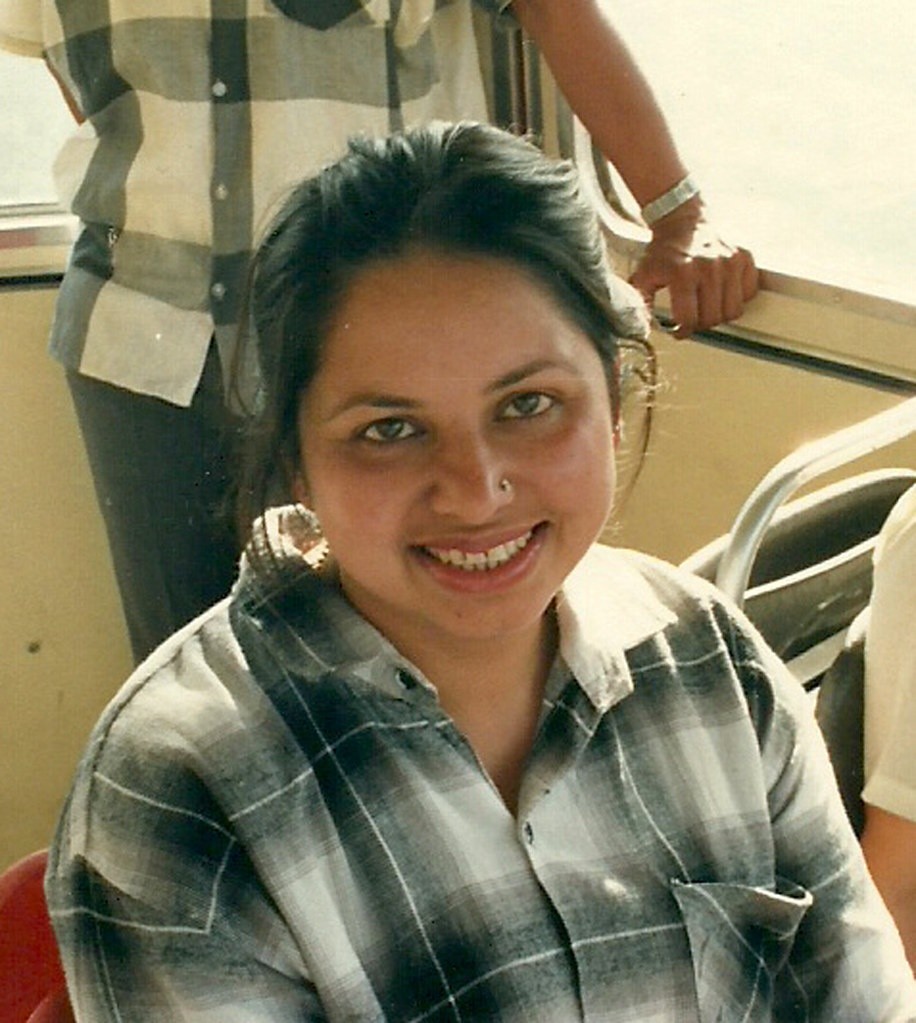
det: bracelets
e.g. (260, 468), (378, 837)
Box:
(640, 173), (700, 225)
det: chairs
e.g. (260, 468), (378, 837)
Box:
(676, 399), (916, 692)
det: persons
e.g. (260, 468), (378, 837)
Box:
(42, 0), (758, 664)
(41, 122), (916, 1023)
